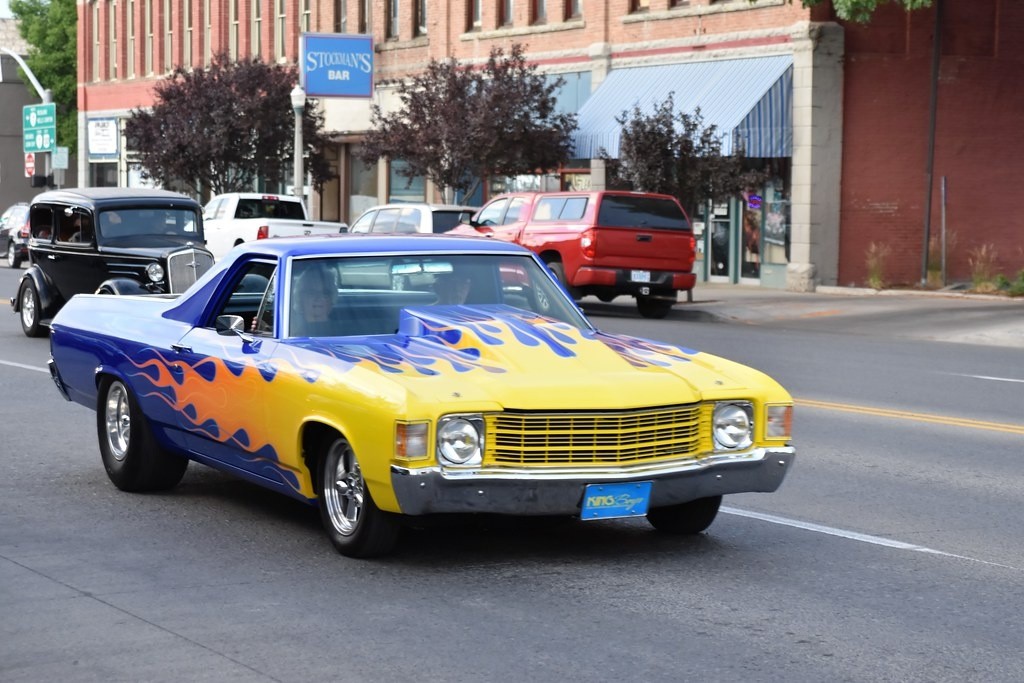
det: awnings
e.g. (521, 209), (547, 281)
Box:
(559, 53), (794, 157)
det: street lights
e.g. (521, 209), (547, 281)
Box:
(290, 83), (306, 197)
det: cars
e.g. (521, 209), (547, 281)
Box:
(0, 201), (30, 269)
(47, 231), (797, 559)
(10, 186), (273, 339)
(334, 205), (480, 292)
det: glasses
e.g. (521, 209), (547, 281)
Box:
(306, 289), (331, 297)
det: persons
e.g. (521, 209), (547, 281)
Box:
(251, 266), (338, 333)
(149, 213), (175, 234)
(417, 263), (472, 307)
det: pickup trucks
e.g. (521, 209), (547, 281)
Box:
(182, 192), (351, 265)
(439, 191), (697, 322)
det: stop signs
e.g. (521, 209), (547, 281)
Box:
(23, 152), (35, 178)
